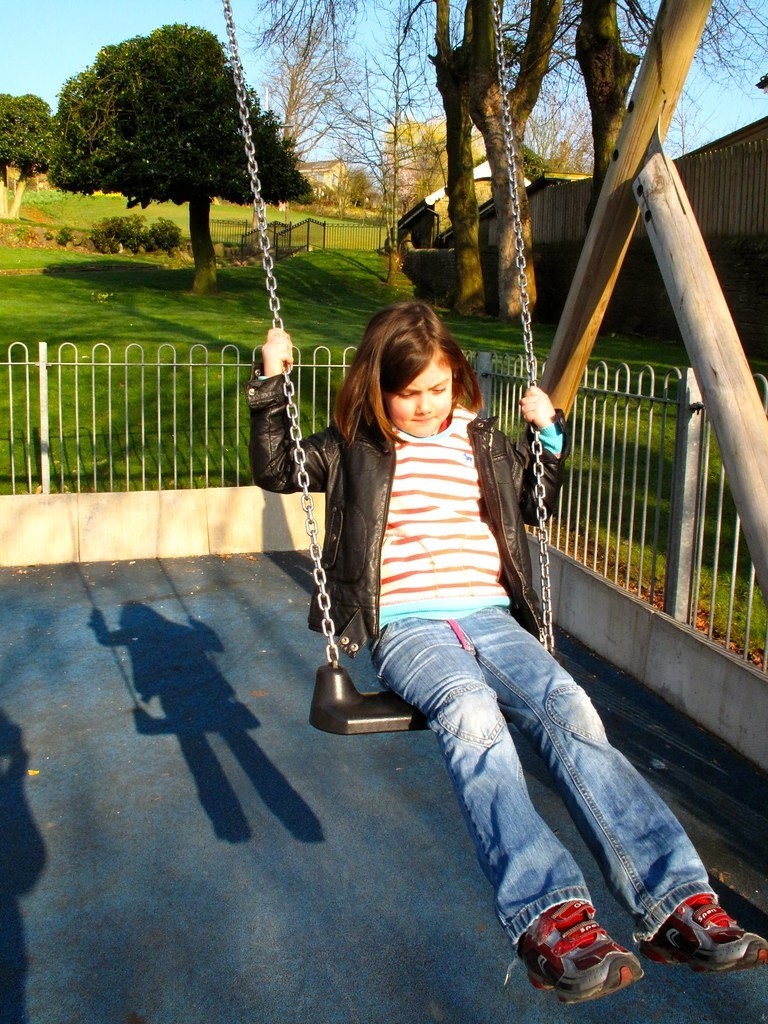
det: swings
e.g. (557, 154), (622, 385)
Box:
(223, 1), (556, 738)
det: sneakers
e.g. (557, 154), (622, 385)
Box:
(633, 892), (768, 973)
(517, 899), (644, 1004)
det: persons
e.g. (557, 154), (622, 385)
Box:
(246, 302), (768, 1006)
